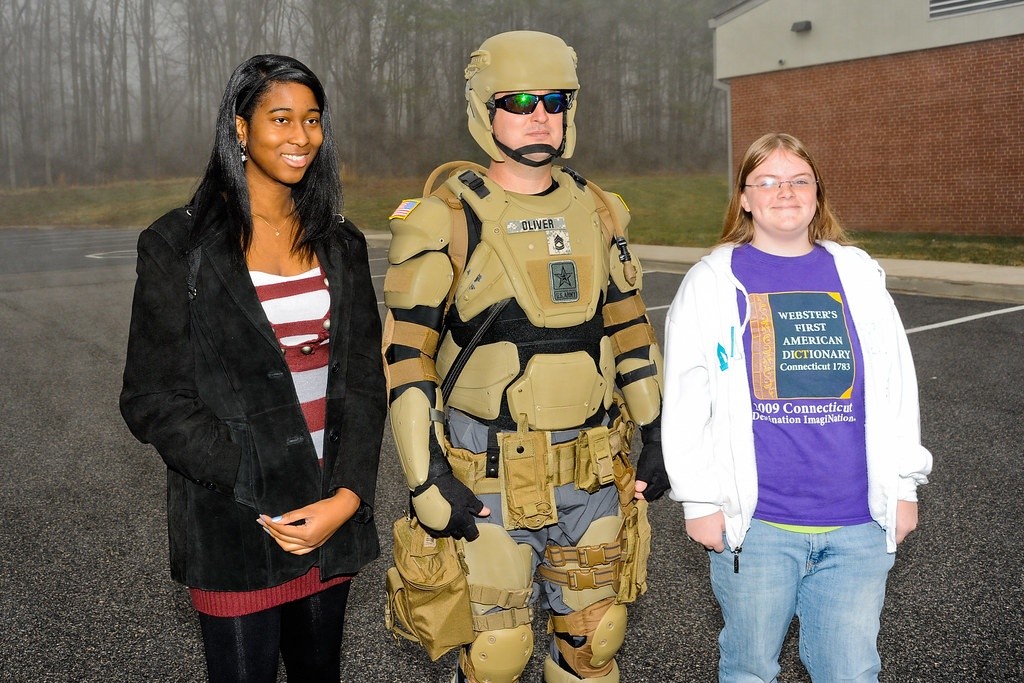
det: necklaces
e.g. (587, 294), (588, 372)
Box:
(251, 199), (294, 236)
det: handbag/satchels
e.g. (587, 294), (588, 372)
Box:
(384, 517), (476, 661)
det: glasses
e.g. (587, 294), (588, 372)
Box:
(494, 91), (567, 114)
(743, 177), (819, 190)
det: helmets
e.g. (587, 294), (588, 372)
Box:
(463, 29), (580, 161)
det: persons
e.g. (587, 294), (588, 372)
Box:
(661, 135), (933, 683)
(118, 55), (386, 683)
(379, 31), (671, 683)
